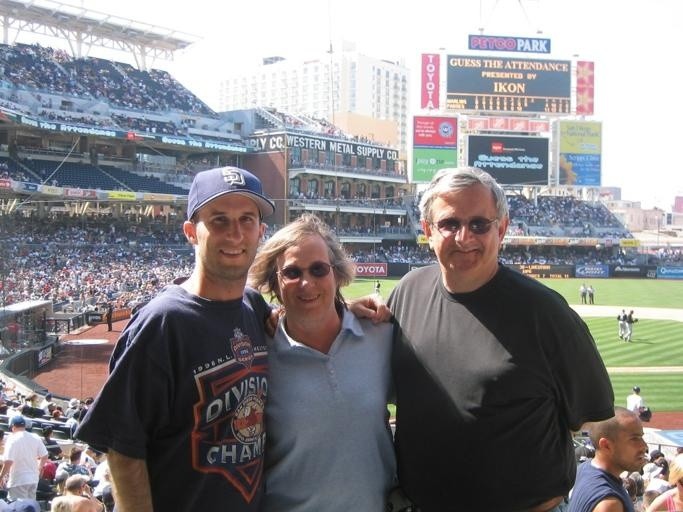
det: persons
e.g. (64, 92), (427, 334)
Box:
(552, 386), (683, 511)
(1, 38), (242, 147)
(288, 186), (438, 264)
(616, 310), (638, 342)
(0, 209), (195, 358)
(73, 167), (394, 511)
(657, 247), (683, 262)
(580, 282), (595, 304)
(385, 164), (614, 512)
(0, 380), (117, 512)
(498, 194), (639, 268)
(0, 160), (59, 188)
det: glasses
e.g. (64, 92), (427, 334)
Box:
(277, 261), (331, 279)
(437, 219), (498, 235)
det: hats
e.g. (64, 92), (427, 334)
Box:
(76, 474), (98, 488)
(643, 463), (663, 478)
(69, 398), (80, 410)
(9, 416), (25, 430)
(187, 165), (274, 222)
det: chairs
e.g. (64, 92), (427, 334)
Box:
(0, 397), (73, 512)
